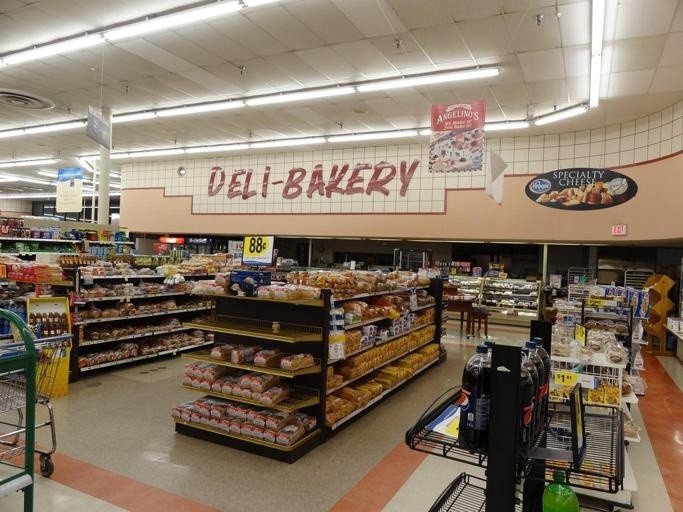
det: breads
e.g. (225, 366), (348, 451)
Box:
(172, 396), (222, 428)
(253, 348), (280, 368)
(221, 374), (288, 404)
(181, 362), (222, 392)
(277, 352), (315, 369)
(210, 343), (261, 364)
(222, 404), (317, 446)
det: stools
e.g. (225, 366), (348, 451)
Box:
(472, 309), (490, 338)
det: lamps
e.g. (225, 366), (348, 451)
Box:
(0, 0), (604, 169)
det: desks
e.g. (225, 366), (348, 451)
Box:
(438, 299), (475, 338)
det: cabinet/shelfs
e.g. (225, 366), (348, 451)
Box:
(5, 268), (76, 379)
(567, 282), (648, 315)
(547, 315), (629, 405)
(446, 274), (480, 305)
(327, 287), (455, 433)
(632, 317), (646, 391)
(481, 278), (541, 317)
(0, 357), (31, 512)
(50, 257), (190, 272)
(514, 350), (630, 509)
(4, 219), (139, 255)
(77, 279), (213, 370)
(405, 348), (498, 508)
(173, 287), (328, 457)
(644, 274), (676, 357)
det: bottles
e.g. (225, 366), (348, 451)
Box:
(272, 322), (280, 334)
(458, 337), (552, 455)
(302, 271), (310, 285)
(0, 303), (25, 334)
(59, 254), (101, 267)
(293, 271), (300, 285)
(542, 471), (580, 512)
(29, 311), (68, 339)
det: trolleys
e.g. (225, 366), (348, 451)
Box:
(0, 334), (74, 477)
